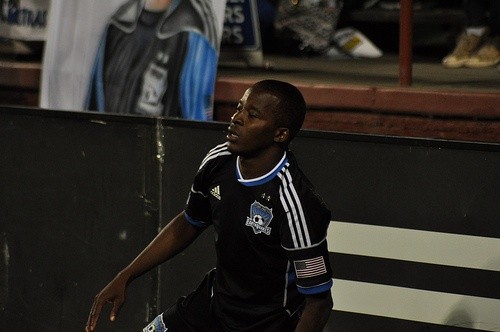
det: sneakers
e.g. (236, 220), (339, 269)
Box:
(464, 39), (500, 68)
(442, 27), (486, 69)
(324, 45), (348, 58)
(331, 26), (383, 58)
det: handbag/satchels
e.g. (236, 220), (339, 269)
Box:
(274, 0), (344, 56)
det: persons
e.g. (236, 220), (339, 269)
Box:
(85, 79), (337, 332)
(256, 0), (499, 69)
